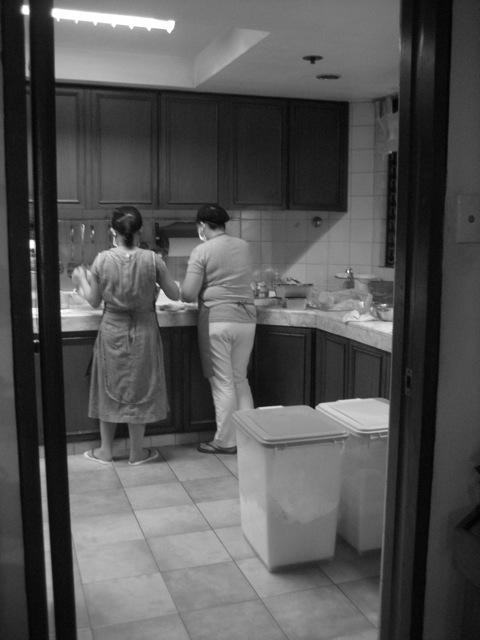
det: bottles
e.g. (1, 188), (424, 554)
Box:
(257, 282), (269, 300)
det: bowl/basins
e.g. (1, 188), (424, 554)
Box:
(375, 304), (394, 322)
(274, 283), (313, 298)
(368, 281), (394, 305)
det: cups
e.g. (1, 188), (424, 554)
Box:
(350, 291), (373, 314)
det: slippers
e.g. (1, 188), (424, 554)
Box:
(128, 447), (159, 465)
(198, 442), (237, 454)
(83, 449), (114, 464)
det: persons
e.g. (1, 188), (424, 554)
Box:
(71, 203), (180, 466)
(184, 199), (260, 454)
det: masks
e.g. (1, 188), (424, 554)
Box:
(197, 227), (206, 241)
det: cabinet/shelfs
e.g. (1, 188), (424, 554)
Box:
(313, 308), (391, 406)
(24, 80), (91, 218)
(225, 94), (350, 215)
(30, 311), (177, 443)
(93, 83), (225, 214)
(182, 300), (218, 432)
(251, 301), (311, 405)
